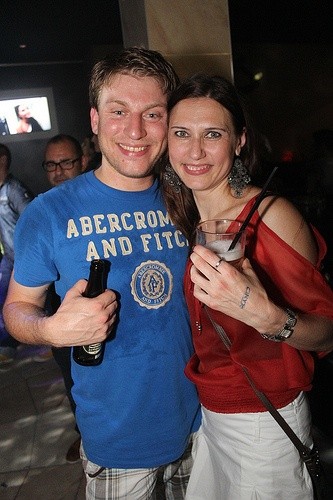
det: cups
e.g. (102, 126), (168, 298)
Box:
(195, 219), (246, 298)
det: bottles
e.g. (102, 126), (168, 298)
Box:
(71, 259), (105, 367)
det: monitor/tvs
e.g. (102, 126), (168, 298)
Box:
(0, 87), (60, 143)
